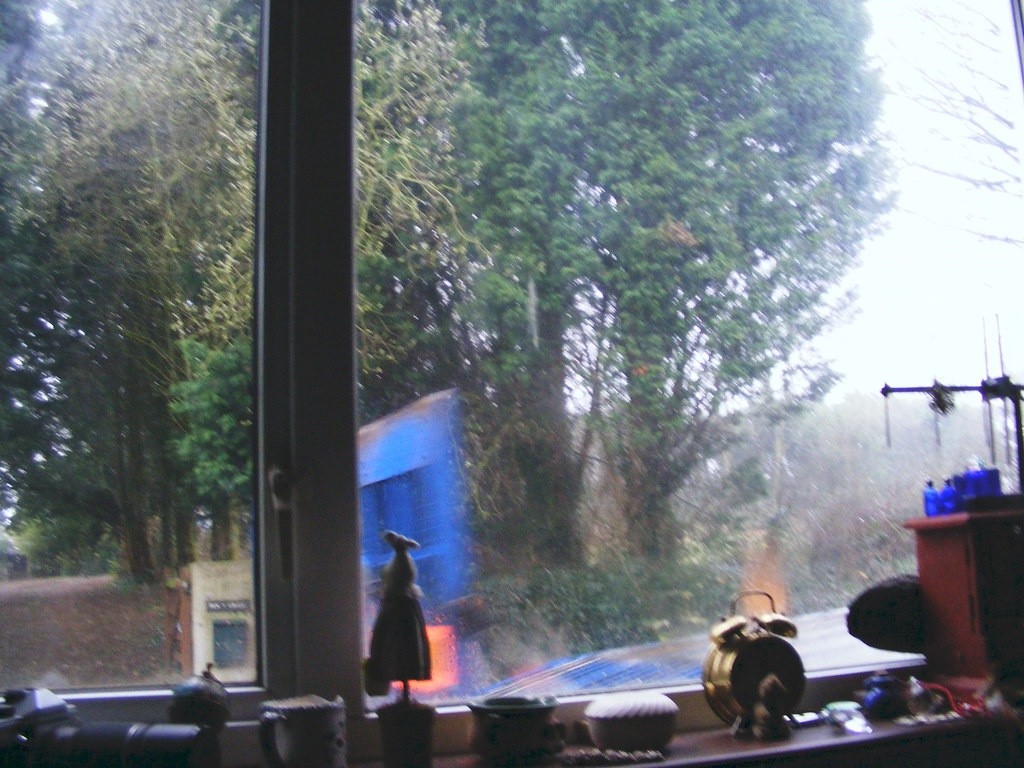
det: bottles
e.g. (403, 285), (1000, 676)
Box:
(942, 480), (955, 514)
(924, 481), (941, 517)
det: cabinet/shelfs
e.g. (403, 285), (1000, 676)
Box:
(901, 506), (1024, 706)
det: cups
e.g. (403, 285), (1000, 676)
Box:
(258, 700), (348, 768)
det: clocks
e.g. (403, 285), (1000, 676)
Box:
(703, 589), (806, 742)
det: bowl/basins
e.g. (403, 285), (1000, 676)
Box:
(584, 691), (678, 749)
